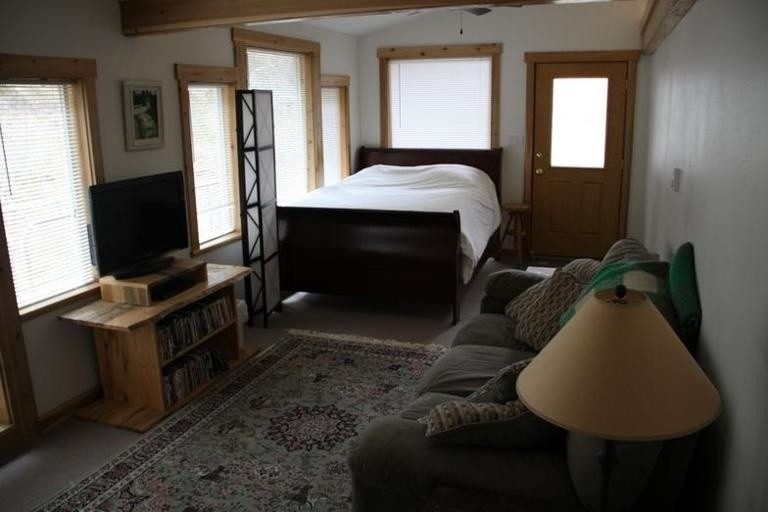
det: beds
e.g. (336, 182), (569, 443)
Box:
(276, 146), (501, 324)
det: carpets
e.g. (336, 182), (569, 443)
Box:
(32, 329), (447, 512)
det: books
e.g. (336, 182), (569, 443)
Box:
(148, 294), (237, 412)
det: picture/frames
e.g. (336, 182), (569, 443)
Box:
(122, 77), (164, 152)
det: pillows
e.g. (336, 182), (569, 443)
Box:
(515, 266), (588, 350)
(506, 258), (606, 315)
(603, 239), (655, 266)
(466, 359), (532, 403)
(418, 401), (529, 436)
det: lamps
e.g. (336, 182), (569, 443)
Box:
(515, 285), (726, 511)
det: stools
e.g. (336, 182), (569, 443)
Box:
(495, 204), (535, 270)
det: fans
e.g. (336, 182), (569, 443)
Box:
(407, 5), (524, 17)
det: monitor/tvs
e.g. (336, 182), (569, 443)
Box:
(87, 170), (189, 281)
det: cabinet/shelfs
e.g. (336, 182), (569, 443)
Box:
(57, 263), (253, 433)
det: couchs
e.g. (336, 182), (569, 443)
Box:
(347, 241), (725, 511)
